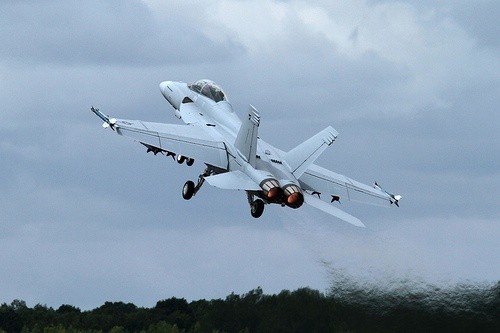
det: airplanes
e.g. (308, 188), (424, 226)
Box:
(91, 77), (401, 230)
